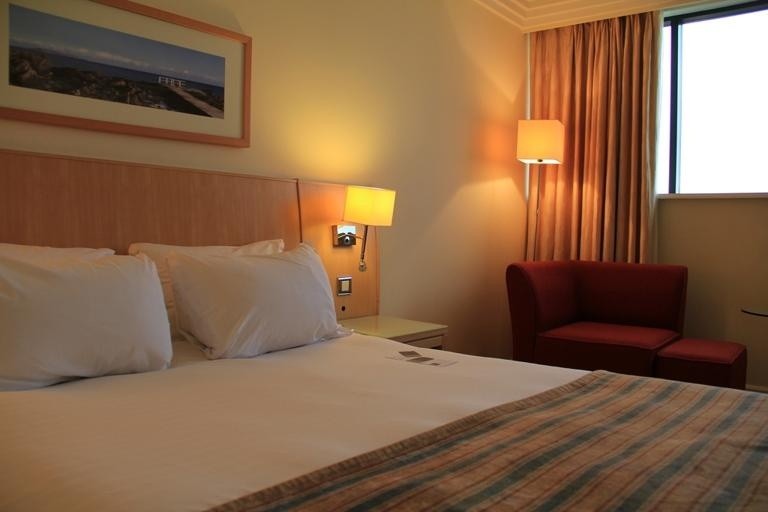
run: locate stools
[657,336,748,389]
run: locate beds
[2,149,766,510]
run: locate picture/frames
[0,0,257,149]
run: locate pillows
[1,248,175,391]
[0,241,116,255]
[125,233,287,344]
[165,240,353,362]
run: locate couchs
[504,259,691,379]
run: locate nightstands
[342,311,452,354]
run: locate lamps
[341,181,398,272]
[515,117,567,263]
